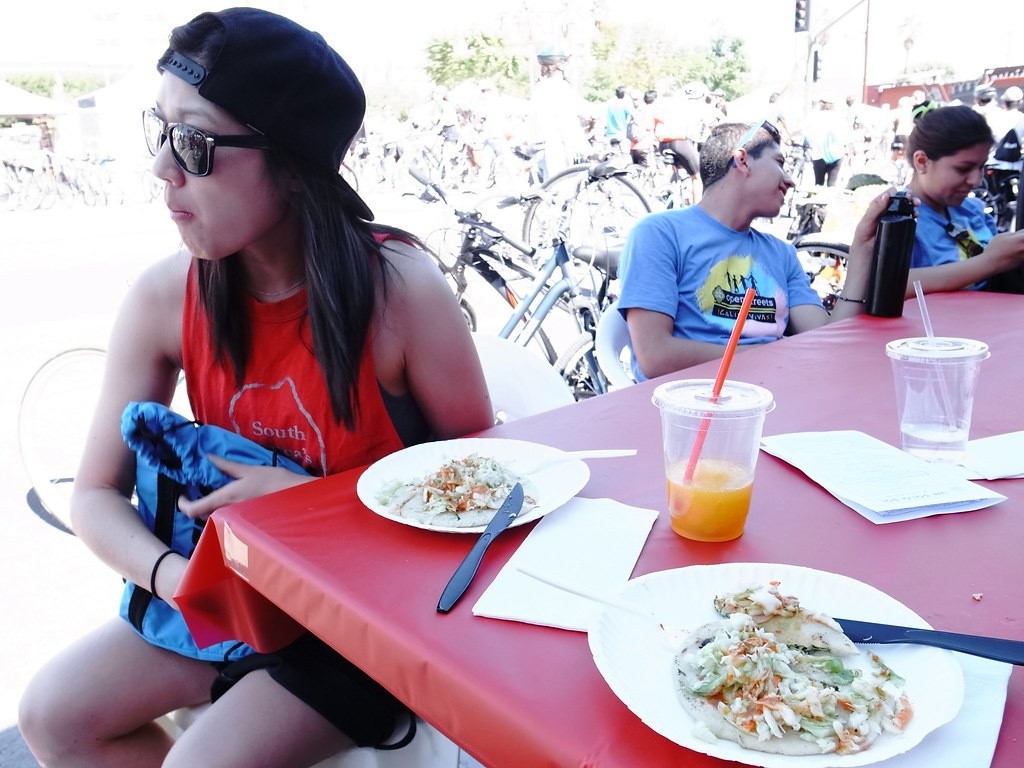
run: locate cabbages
[682,581,902,752]
[415,456,511,519]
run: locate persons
[12,6,493,768]
[613,123,922,381]
[609,87,927,185]
[892,102,1024,299]
[966,84,1024,226]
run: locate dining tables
[177,289,1024,768]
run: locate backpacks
[994,115,1021,162]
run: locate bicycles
[2,106,1024,404]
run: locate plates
[356,436,591,534]
[587,562,964,768]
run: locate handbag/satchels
[120,399,314,663]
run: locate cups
[651,377,776,544]
[884,335,991,468]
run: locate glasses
[142,109,279,177]
[732,121,782,166]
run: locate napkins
[476,497,660,633]
[966,430,1024,482]
[861,650,1013,768]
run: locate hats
[1000,86,1023,102]
[159,6,374,219]
[975,85,996,100]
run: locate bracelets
[839,295,866,305]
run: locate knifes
[832,617,1024,667]
[436,481,525,614]
